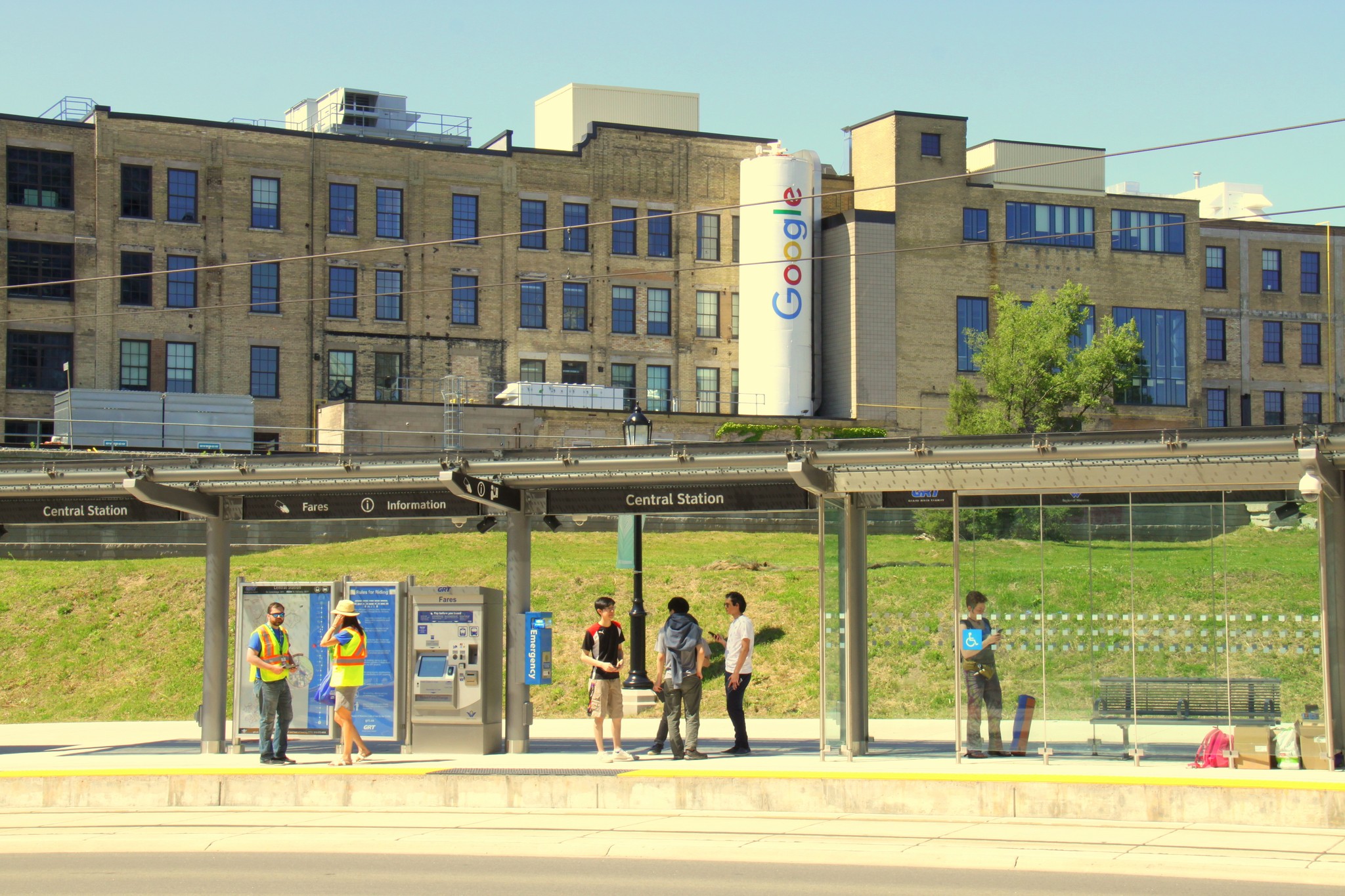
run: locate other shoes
[673,751,685,759]
[720,746,738,754]
[648,749,662,755]
[735,747,751,756]
[275,756,296,764]
[260,758,284,765]
[684,749,708,760]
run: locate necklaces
[968,616,981,625]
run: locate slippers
[327,758,353,766]
[353,752,372,763]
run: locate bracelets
[332,626,336,629]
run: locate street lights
[621,399,653,690]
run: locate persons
[714,591,755,756]
[959,591,1004,759]
[653,597,708,760]
[647,613,712,755]
[581,597,639,762]
[246,603,297,765]
[320,600,372,767]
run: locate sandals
[967,749,988,758]
[988,748,1012,756]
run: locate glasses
[270,612,285,617]
[602,606,616,611]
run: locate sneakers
[611,750,639,761]
[596,751,613,763]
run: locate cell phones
[708,632,719,639]
[997,629,1003,634]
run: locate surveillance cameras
[451,517,466,527]
[572,514,587,525]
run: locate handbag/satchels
[1272,728,1302,769]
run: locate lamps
[477,517,496,535]
[543,514,563,534]
[452,517,467,527]
[572,515,587,526]
[712,347,717,355]
[1298,466,1323,502]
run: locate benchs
[1090,676,1281,760]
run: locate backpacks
[1197,728,1235,767]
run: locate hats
[330,600,360,617]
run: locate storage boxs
[1230,724,1272,770]
[1294,719,1329,770]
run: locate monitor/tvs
[417,656,447,678]
